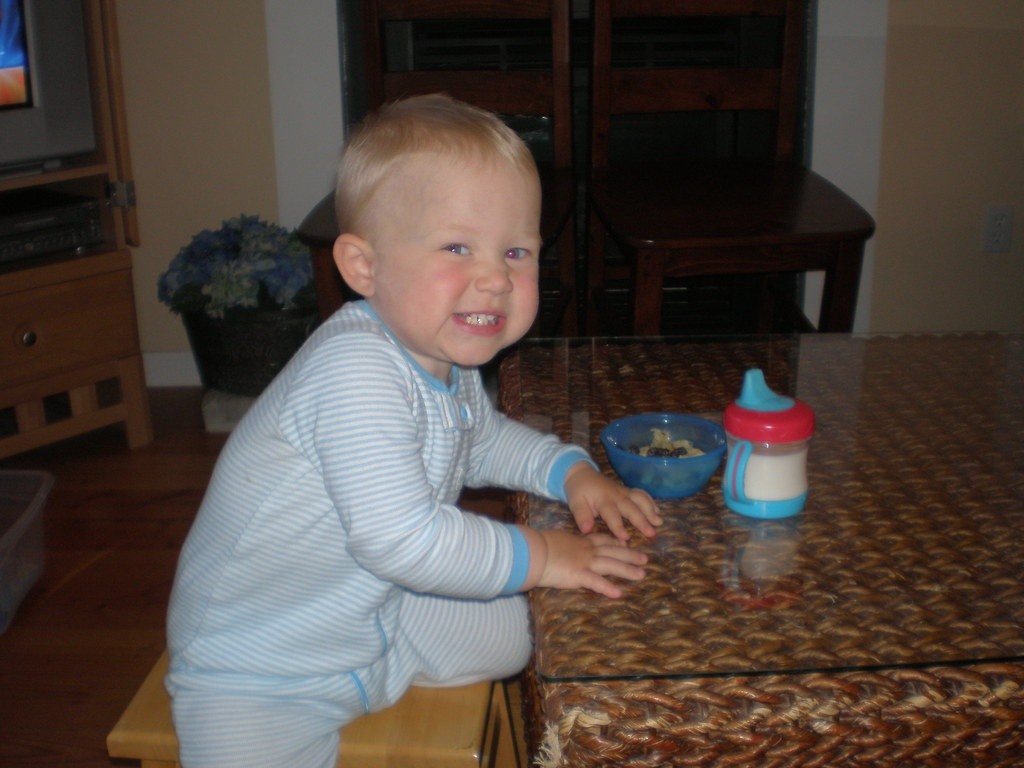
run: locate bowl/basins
[599,413,728,499]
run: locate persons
[165,93,663,768]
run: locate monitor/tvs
[0,0,97,181]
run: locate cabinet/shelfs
[0,0,154,464]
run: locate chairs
[288,0,575,337]
[585,0,878,339]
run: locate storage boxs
[0,463,59,636]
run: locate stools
[107,641,522,768]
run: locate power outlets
[981,205,1016,256]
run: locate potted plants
[156,210,310,399]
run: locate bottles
[722,369,814,518]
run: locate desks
[496,322,1024,768]
